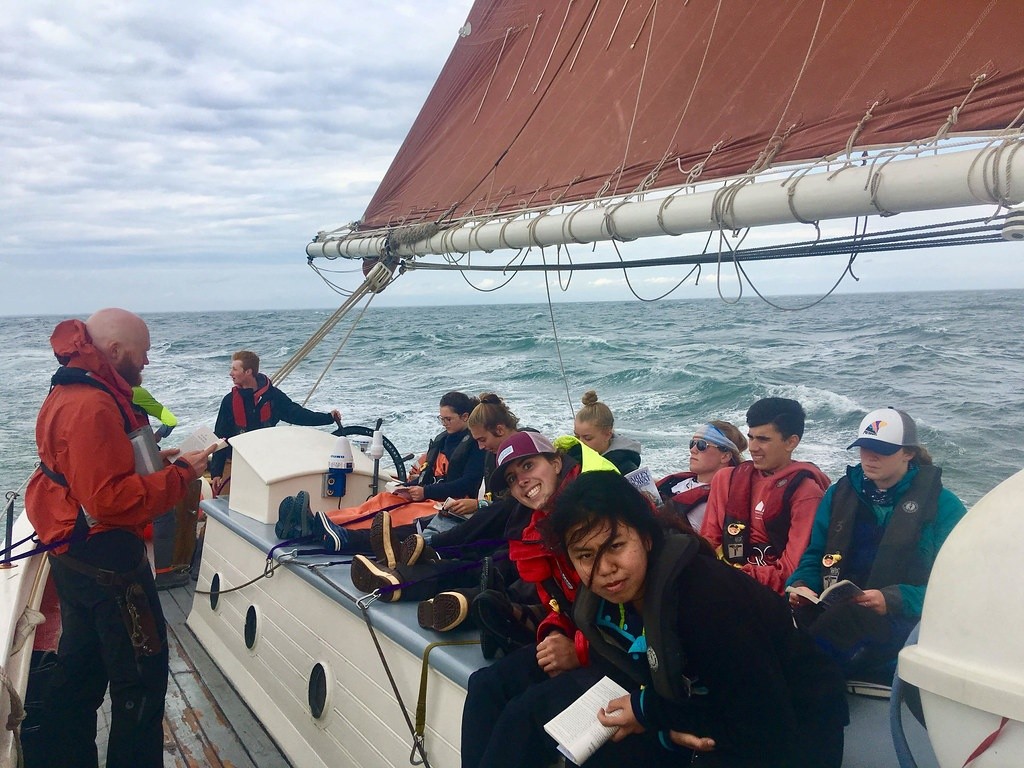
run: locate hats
[490,430,558,492]
[846,406,919,456]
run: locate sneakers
[369,511,402,571]
[418,591,468,632]
[401,534,440,565]
[351,555,406,603]
[154,571,189,591]
[316,511,350,553]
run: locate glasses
[689,439,726,452]
[437,414,462,425]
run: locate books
[167,426,227,466]
[433,497,472,520]
[623,465,664,508]
[386,482,411,501]
[784,579,865,611]
[543,676,632,766]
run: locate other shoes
[293,490,315,540]
[477,590,539,647]
[476,557,507,659]
[275,495,297,539]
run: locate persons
[210,350,341,500]
[23,308,216,768]
[131,386,177,443]
[278,391,967,768]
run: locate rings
[865,602,867,607]
